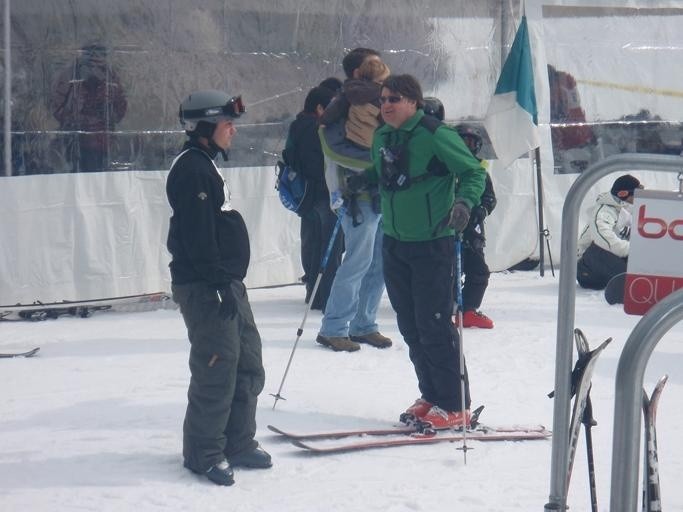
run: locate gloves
[447,202,470,234]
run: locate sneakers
[185,452,234,483]
[349,333,393,348]
[456,309,493,329]
[229,445,272,468]
[406,398,471,430]
[316,334,360,352]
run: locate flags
[484,12,543,168]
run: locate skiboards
[642,375,668,512]
[562,328,612,512]
[267,425,554,452]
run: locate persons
[346,73,488,433]
[167,88,274,485]
[281,48,395,350]
[548,64,591,165]
[417,95,493,330]
[53,33,128,172]
[577,174,644,290]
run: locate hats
[611,175,643,199]
[358,56,392,84]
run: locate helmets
[422,97,443,120]
[179,89,235,133]
[454,124,482,156]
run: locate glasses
[179,94,247,118]
[378,94,405,105]
